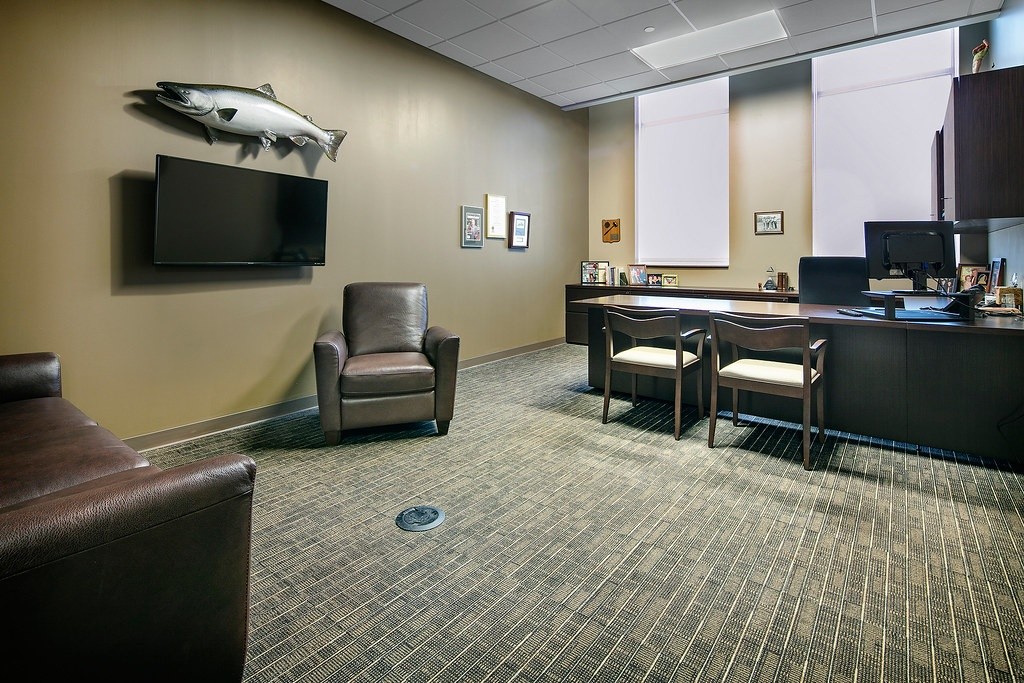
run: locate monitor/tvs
[864,221,956,294]
[153,154,328,267]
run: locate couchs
[0,350,256,683]
[313,281,460,445]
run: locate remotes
[837,308,863,317]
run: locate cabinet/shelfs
[564,283,798,346]
[931,64,1024,235]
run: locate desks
[571,295,1024,477]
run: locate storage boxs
[995,287,1022,305]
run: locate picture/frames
[647,273,662,286]
[461,204,485,248]
[581,261,610,284]
[986,257,1005,293]
[956,263,990,294]
[937,273,958,293]
[753,210,784,235]
[662,274,679,287]
[508,211,531,249]
[627,263,648,285]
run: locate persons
[466,218,479,241]
[648,275,662,284]
[962,267,987,290]
[761,216,778,230]
[633,268,643,284]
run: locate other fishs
[156,81,348,163]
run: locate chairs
[601,305,708,441]
[797,256,905,308]
[706,311,828,471]
